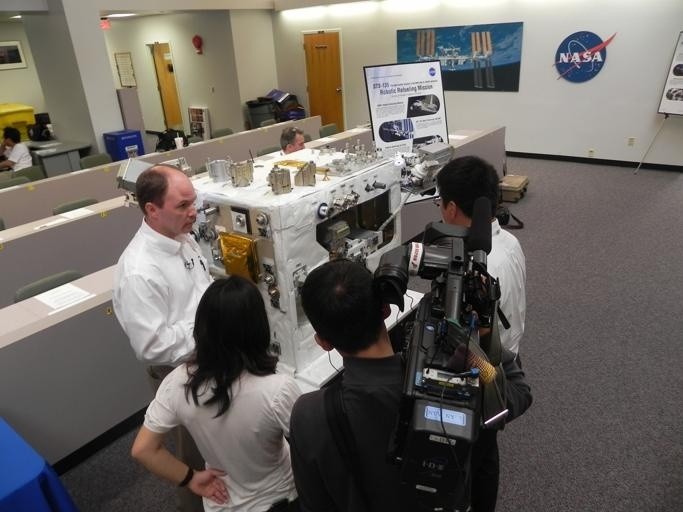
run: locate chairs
[0,118,342,303]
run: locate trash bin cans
[246,100,280,129]
[103,130,144,162]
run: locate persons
[431,156,526,511]
[280,127,305,156]
[131,275,305,511]
[112,164,216,512]
[0,128,33,172]
[288,258,532,512]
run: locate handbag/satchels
[27,123,50,141]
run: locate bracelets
[179,467,194,488]
